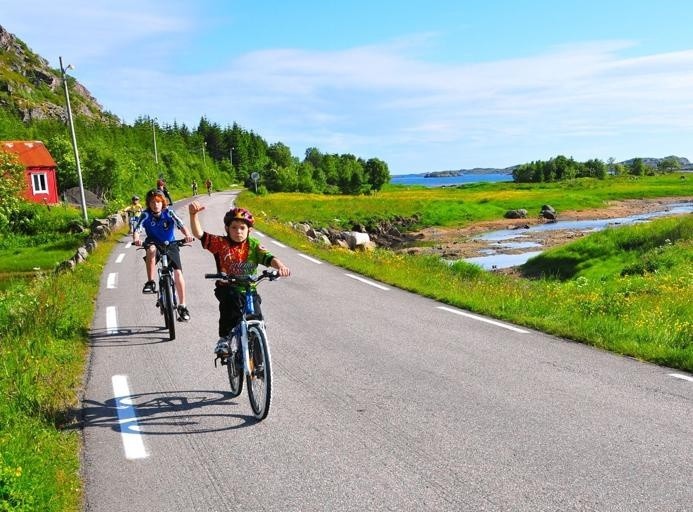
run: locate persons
[155,175,169,196]
[132,188,194,322]
[191,179,197,196]
[187,200,291,381]
[204,178,212,192]
[122,194,142,235]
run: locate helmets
[224,207,255,227]
[146,188,166,205]
[132,195,139,201]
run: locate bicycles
[131,238,195,340]
[193,189,198,196]
[207,185,212,196]
[124,210,140,233]
[204,270,291,421]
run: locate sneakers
[214,339,230,354]
[176,306,190,320]
[143,281,156,294]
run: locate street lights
[202,142,208,165]
[59,56,89,223]
[229,147,235,165]
[152,116,158,163]
